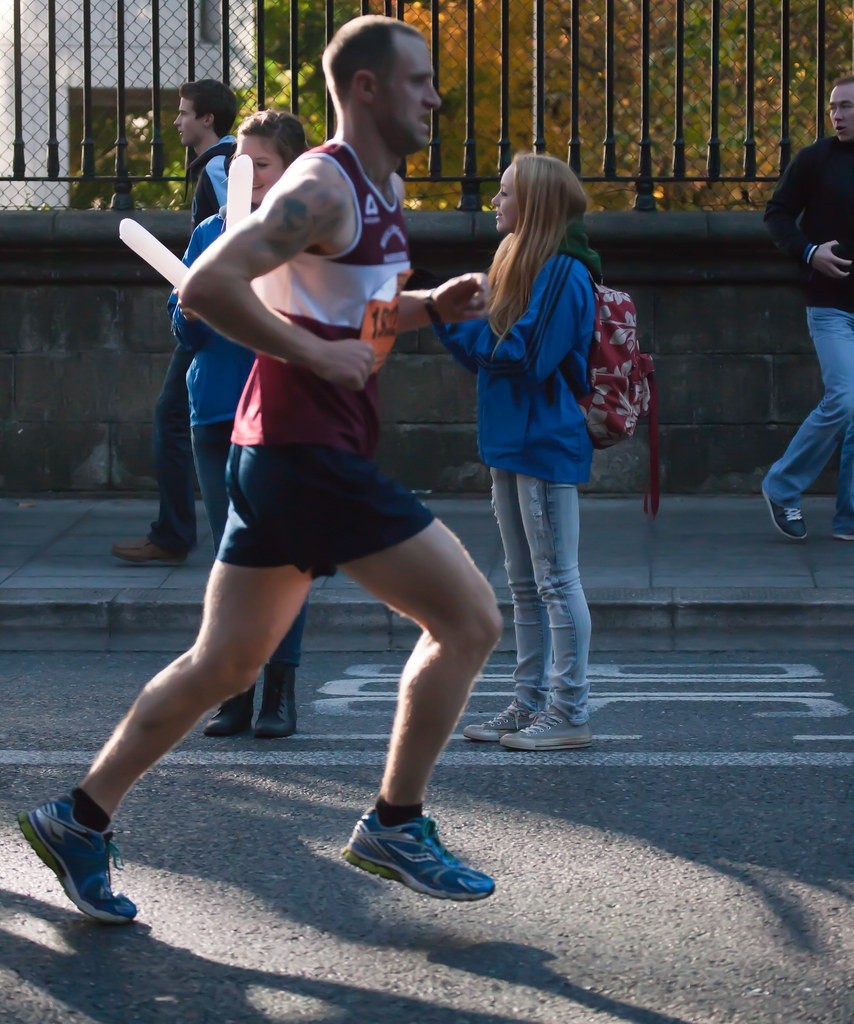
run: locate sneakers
[340,806,495,901]
[762,482,807,539]
[18,794,137,923]
[500,704,592,750]
[833,534,854,540]
[463,699,540,741]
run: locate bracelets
[423,288,444,329]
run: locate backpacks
[562,268,655,450]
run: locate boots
[203,683,255,736]
[255,661,297,736]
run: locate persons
[761,76,854,539]
[402,152,603,749]
[14,14,504,924]
[166,111,314,739]
[110,81,237,565]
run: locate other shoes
[112,537,188,565]
